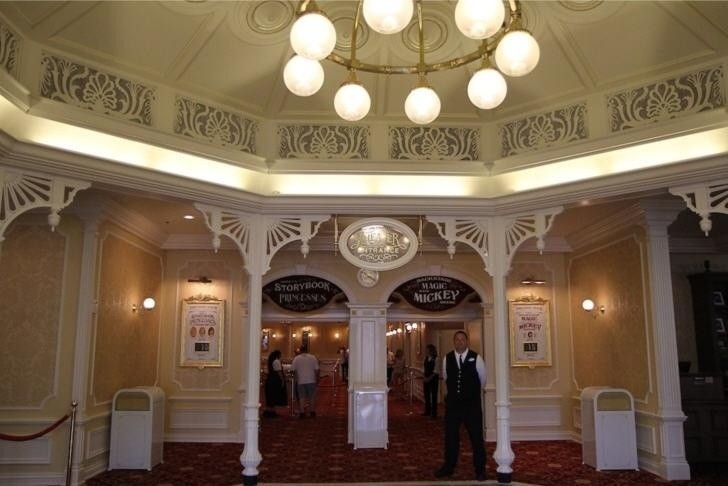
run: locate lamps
[282,0,539,125]
[188,277,212,283]
[522,279,547,285]
[132,298,155,312]
[582,300,605,314]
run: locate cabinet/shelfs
[686,260,728,375]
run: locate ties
[458,354,462,370]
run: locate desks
[681,374,728,470]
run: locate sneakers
[475,470,487,481]
[433,465,454,478]
[299,410,315,418]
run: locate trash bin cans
[581,386,640,472]
[107,386,162,471]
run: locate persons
[420,344,440,418]
[433,330,489,482]
[262,350,288,418]
[333,344,348,384]
[386,346,396,385]
[391,349,405,385]
[290,346,320,419]
